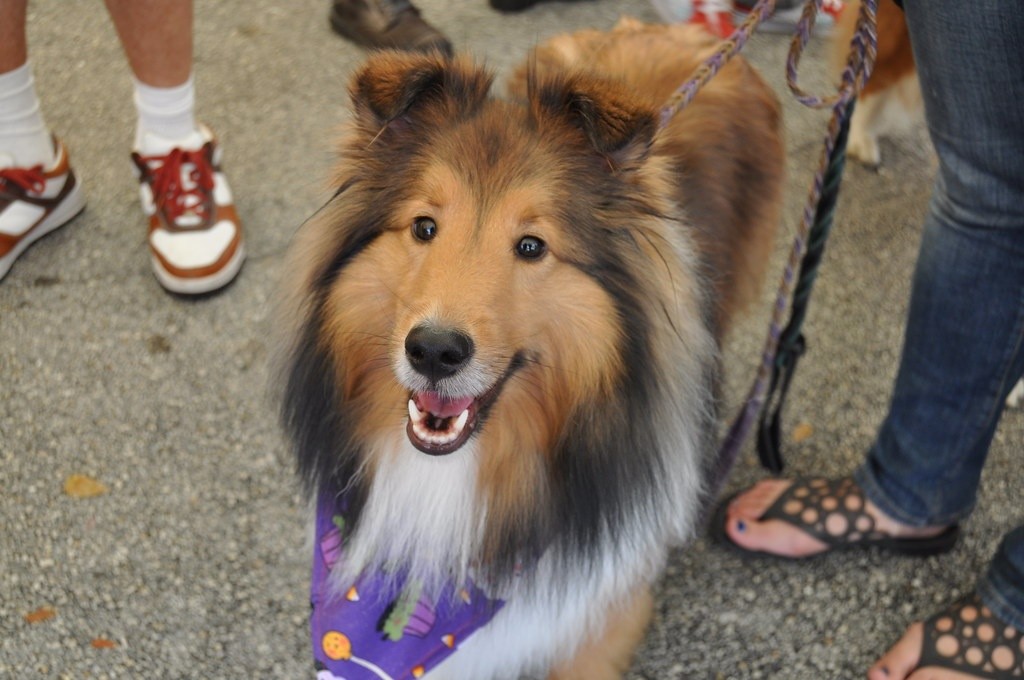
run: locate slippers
[709,474,961,559]
[864,589,1023,680]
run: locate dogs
[827,1,1024,411]
[258,11,784,680]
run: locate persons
[1,0,248,295]
[704,0,1024,680]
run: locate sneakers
[1,132,84,285]
[731,5,836,35]
[130,115,248,293]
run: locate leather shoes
[331,0,453,60]
